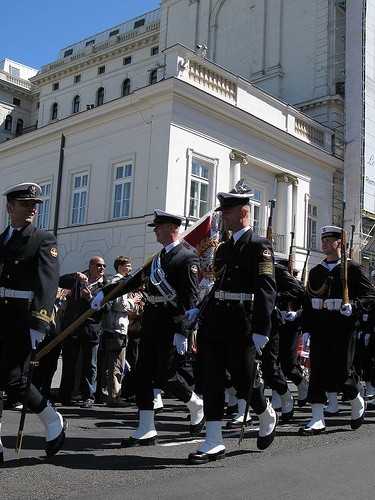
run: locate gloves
[284,311,297,322]
[365,334,370,346]
[253,333,269,355]
[173,333,188,355]
[340,303,352,316]
[185,308,199,320]
[30,329,46,349]
[91,290,104,311]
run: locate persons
[3,192,375,462]
[0,183,68,463]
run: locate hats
[319,226,347,239]
[147,209,184,227]
[1,182,44,204]
[214,192,254,212]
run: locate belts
[0,287,35,299]
[215,290,254,303]
[147,295,169,302]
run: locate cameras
[80,278,88,282]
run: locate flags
[183,208,225,306]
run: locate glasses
[94,263,107,268]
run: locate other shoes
[121,435,159,448]
[257,410,279,451]
[45,412,68,457]
[190,416,205,438]
[188,448,226,465]
[224,386,375,436]
[14,396,137,413]
[137,405,164,416]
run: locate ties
[160,248,166,258]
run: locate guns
[15,286,71,453]
[349,223,356,261]
[288,213,296,277]
[341,200,350,312]
[264,199,275,246]
[301,249,312,288]
